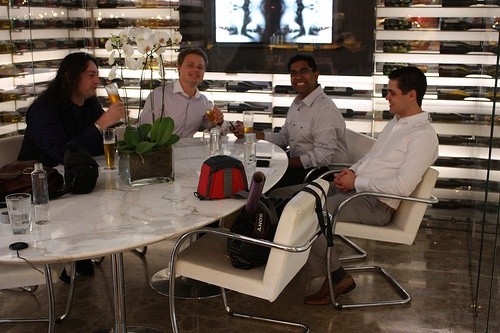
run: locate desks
[0,131,289,333]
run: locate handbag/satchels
[226,196,291,270]
[64,139,99,194]
[193,154,249,200]
[0,159,65,210]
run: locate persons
[140,47,228,138]
[17,52,125,278]
[266,66,438,304]
[231,56,349,197]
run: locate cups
[243,142,257,184]
[103,128,117,170]
[243,110,254,143]
[210,128,222,156]
[5,193,33,235]
[222,128,229,156]
[105,82,127,119]
[203,91,217,126]
[203,133,214,161]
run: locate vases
[114,142,176,187]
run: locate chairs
[0,135,75,323]
[168,178,332,333]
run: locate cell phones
[256,160,270,168]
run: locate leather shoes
[304,272,357,305]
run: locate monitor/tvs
[211,0,338,49]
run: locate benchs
[322,128,439,309]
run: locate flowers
[105,25,182,153]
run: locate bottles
[273,106,290,115]
[31,163,51,224]
[0,0,179,141]
[336,109,366,118]
[274,84,298,94]
[374,0,500,194]
[227,102,268,112]
[324,85,367,96]
[226,79,267,92]
[196,80,223,90]
